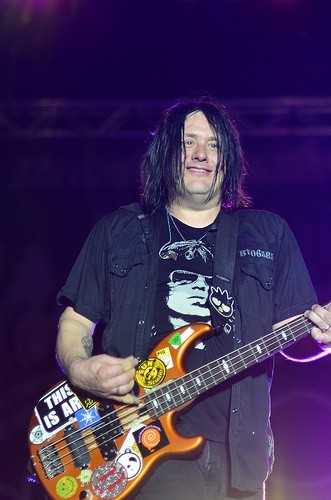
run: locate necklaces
[164,204,210,260]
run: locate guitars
[27,302,331,500]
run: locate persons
[53,97,331,500]
